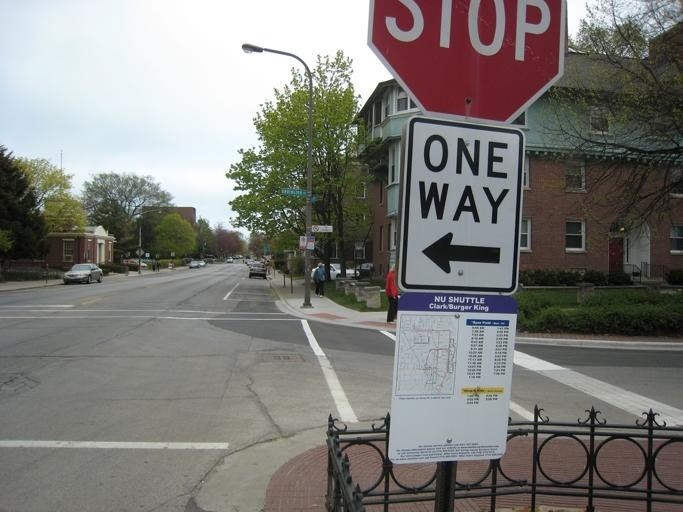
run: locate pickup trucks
[204,255,216,264]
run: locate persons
[312,263,327,298]
[155,262,161,272]
[384,263,399,324]
[152,258,156,272]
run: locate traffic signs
[395,116,525,295]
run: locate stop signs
[367,0,568,126]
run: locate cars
[121,259,146,271]
[64,263,103,284]
[189,261,205,269]
[227,255,243,263]
[245,259,266,279]
[311,262,373,279]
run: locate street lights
[171,251,176,269]
[138,209,162,274]
[242,44,313,308]
